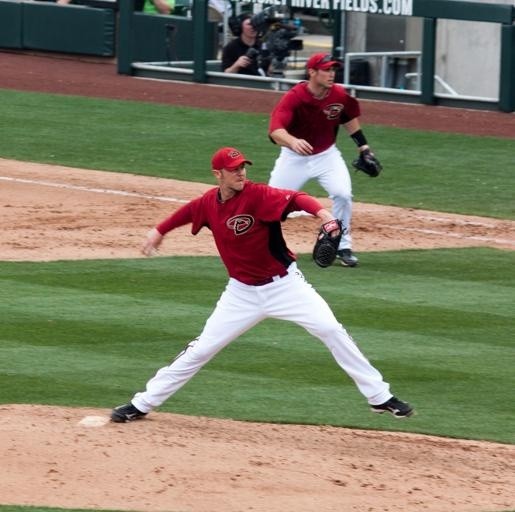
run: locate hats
[305,52,345,72]
[211,146,253,173]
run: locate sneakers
[109,399,148,423]
[369,393,414,420]
[335,248,360,267]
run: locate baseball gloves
[312,219,342,267]
[352,148,382,176]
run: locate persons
[111,147,414,423]
[221,13,273,76]
[266,54,369,267]
[144,0,176,16]
